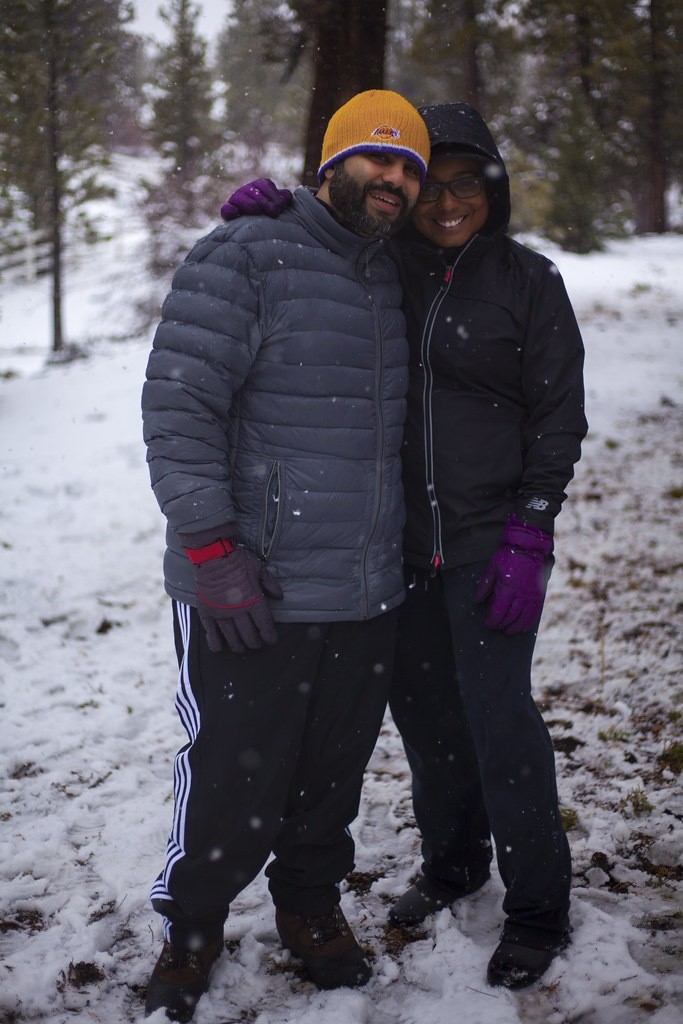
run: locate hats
[316,89,430,191]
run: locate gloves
[220,179,291,221]
[178,521,282,654]
[474,515,553,635]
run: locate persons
[140,89,588,1022]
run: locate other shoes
[486,919,570,994]
[145,928,225,1022]
[276,902,372,992]
[388,876,488,926]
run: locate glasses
[417,176,484,203]
